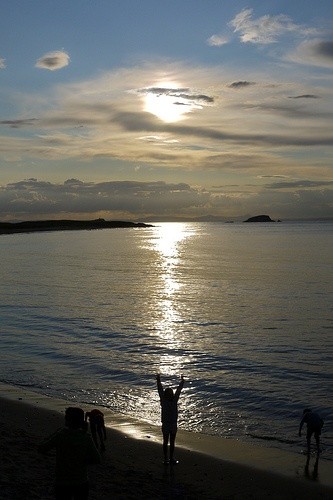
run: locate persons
[45,407,103,500]
[156,372,184,465]
[299,408,323,449]
